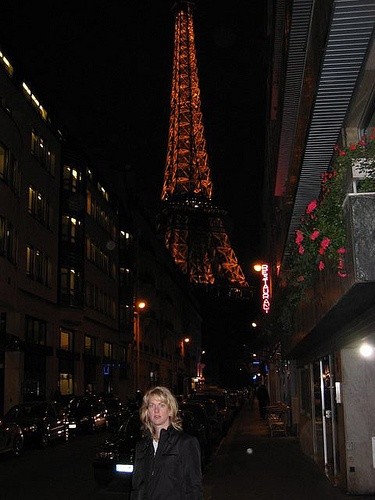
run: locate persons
[128,385,206,500]
[247,383,270,419]
[53,386,62,403]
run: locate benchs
[263,401,290,438]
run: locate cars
[0,417,25,457]
[4,401,73,450]
[96,388,249,487]
[59,394,109,434]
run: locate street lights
[135,299,146,391]
[181,336,190,395]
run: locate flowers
[267,128,374,293]
